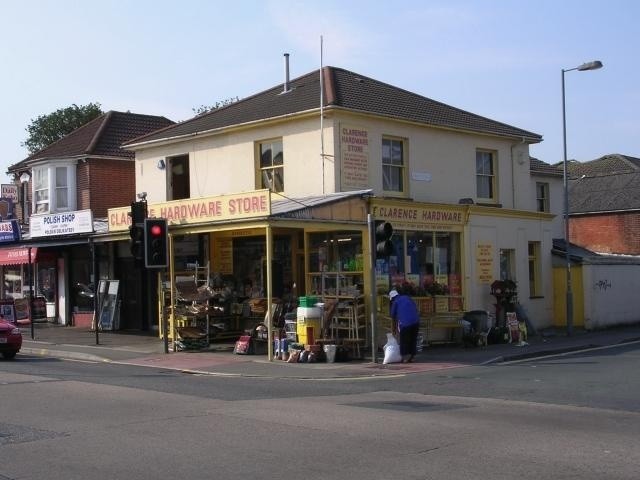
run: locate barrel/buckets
[323,344,336,362]
[464,310,488,348]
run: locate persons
[388,289,421,364]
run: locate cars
[0,315,23,361]
[75,282,96,310]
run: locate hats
[388,290,401,301]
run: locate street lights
[560,59,604,336]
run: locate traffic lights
[374,219,394,259]
[144,218,170,268]
[128,202,147,260]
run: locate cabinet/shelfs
[156,267,367,359]
